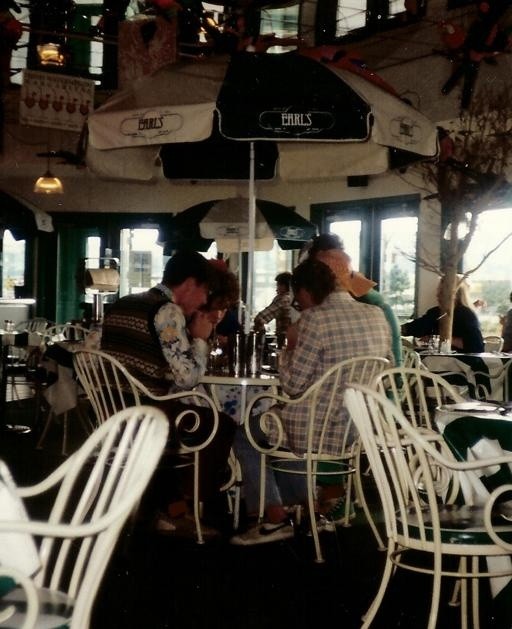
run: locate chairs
[482,335,505,353]
[371,366,467,449]
[3,315,105,460]
[242,357,395,565]
[469,358,512,403]
[0,403,168,629]
[402,345,471,410]
[363,348,422,476]
[70,345,243,545]
[342,377,511,629]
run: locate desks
[432,400,511,607]
[0,458,42,606]
[191,373,282,531]
[414,351,512,406]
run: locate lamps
[32,127,66,196]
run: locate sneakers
[229,519,295,546]
[157,510,218,536]
[302,492,355,538]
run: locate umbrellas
[153,198,316,327]
[1,189,57,242]
[80,51,444,345]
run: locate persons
[498,291,512,403]
[400,273,492,400]
[98,233,405,545]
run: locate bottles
[3,319,16,334]
[428,334,451,355]
[229,330,263,376]
